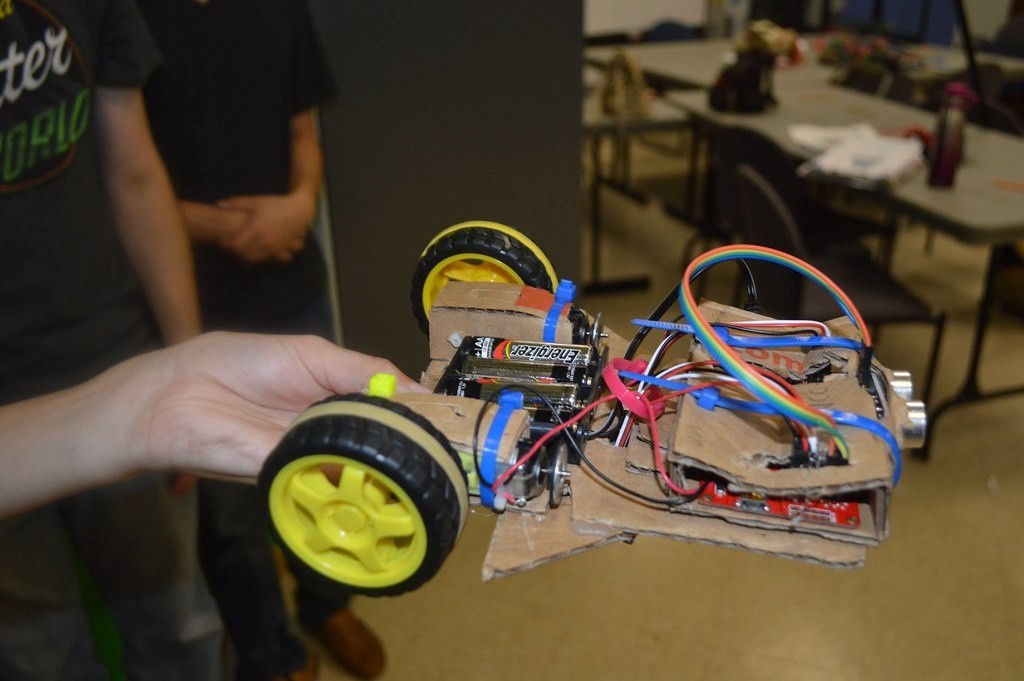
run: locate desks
[575,33,1024,468]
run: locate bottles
[930,81,966,189]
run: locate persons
[0,330,432,521]
[87,1,386,681]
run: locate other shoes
[290,647,318,681]
[296,609,386,681]
